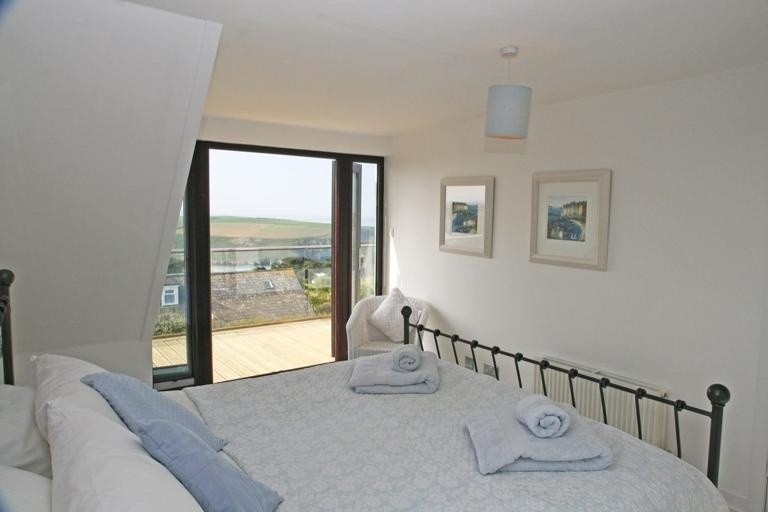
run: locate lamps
[485,47,532,140]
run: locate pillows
[139,419,284,511]
[368,288,421,343]
[27,353,131,445]
[81,372,227,454]
[2,383,52,477]
[0,465,51,512]
[42,401,203,512]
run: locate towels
[515,394,570,438]
[465,402,613,476]
[390,344,421,373]
[347,351,439,394]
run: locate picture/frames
[439,176,494,259]
[529,170,612,272]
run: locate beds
[0,269,733,512]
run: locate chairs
[345,295,430,360]
